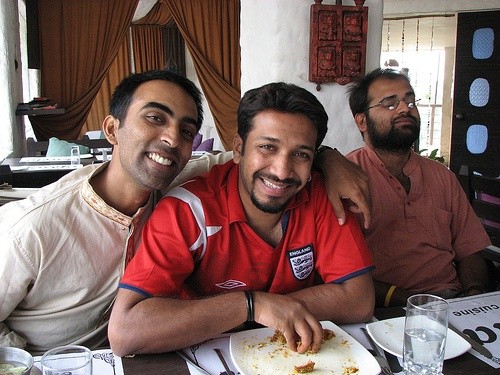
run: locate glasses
[362,95,420,110]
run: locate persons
[104,82,375,358]
[0,70,371,358]
[344,67,491,308]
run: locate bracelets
[315,146,338,164]
[244,290,254,321]
[384,285,396,307]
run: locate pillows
[46,136,89,158]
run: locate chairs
[25,135,89,156]
[87,136,113,154]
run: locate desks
[1,156,102,184]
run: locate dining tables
[33,282,500,375]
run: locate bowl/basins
[0,346,34,375]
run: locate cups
[41,345,93,375]
[402,294,449,375]
[70,147,80,167]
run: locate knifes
[446,322,492,359]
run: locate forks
[359,327,394,375]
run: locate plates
[229,320,382,375]
[366,315,471,360]
